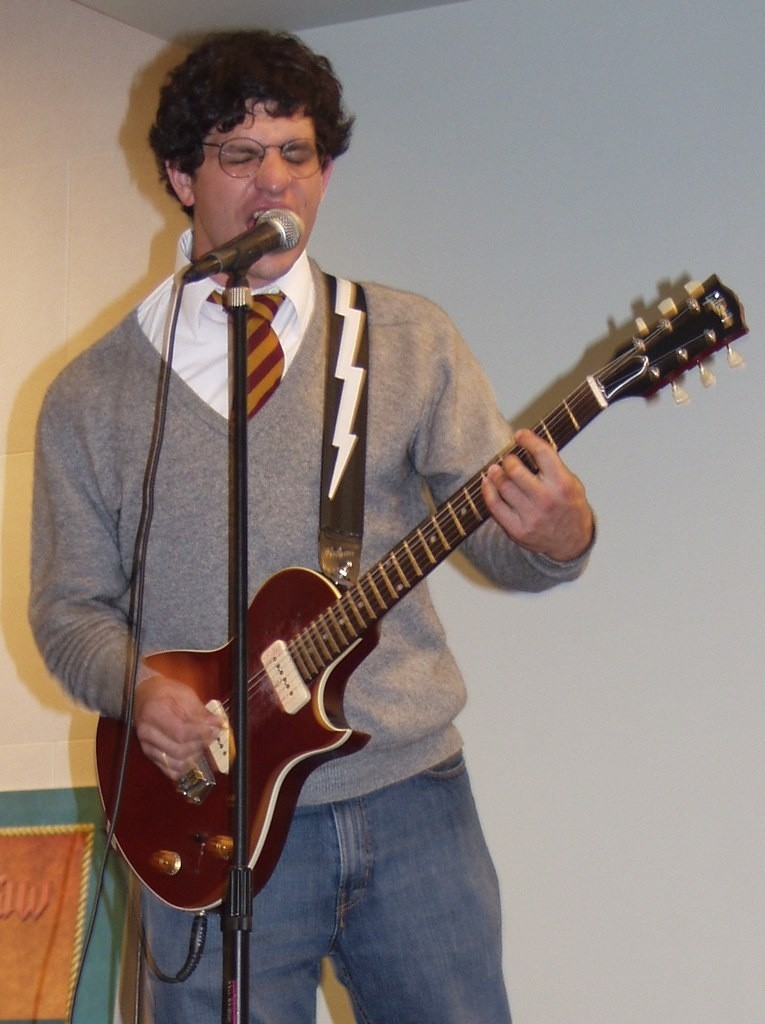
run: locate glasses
[199,137,329,179]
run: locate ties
[207,290,287,422]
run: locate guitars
[92,272,745,916]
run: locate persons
[30,32,597,1023]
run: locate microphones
[184,206,306,283]
